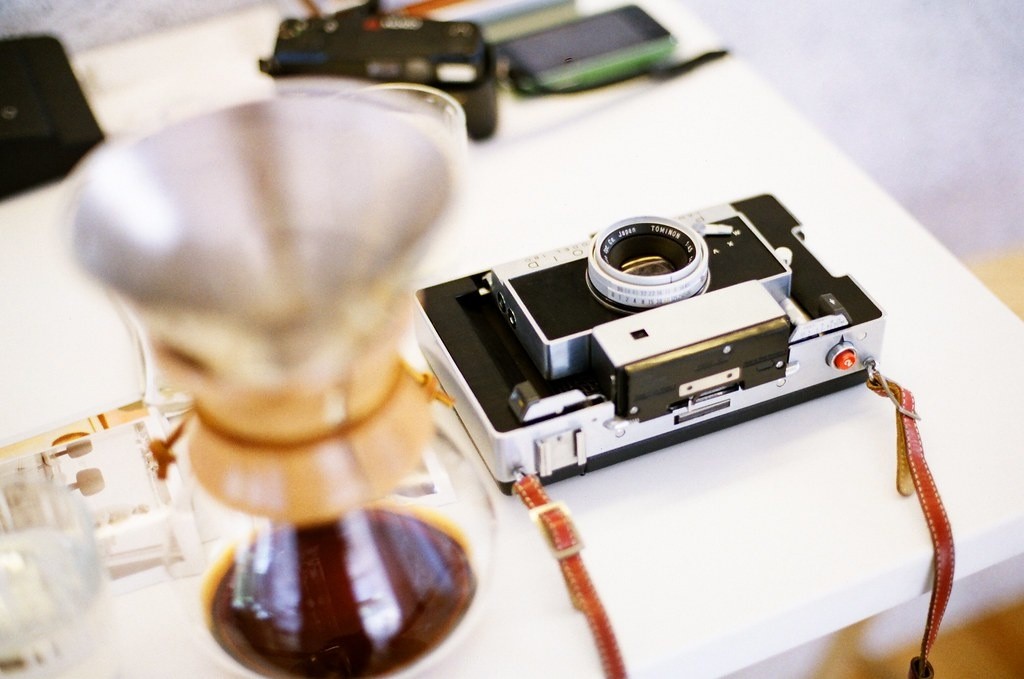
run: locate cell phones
[492,4,679,94]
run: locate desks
[0,0,1023,678]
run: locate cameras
[259,15,497,142]
[414,192,888,497]
